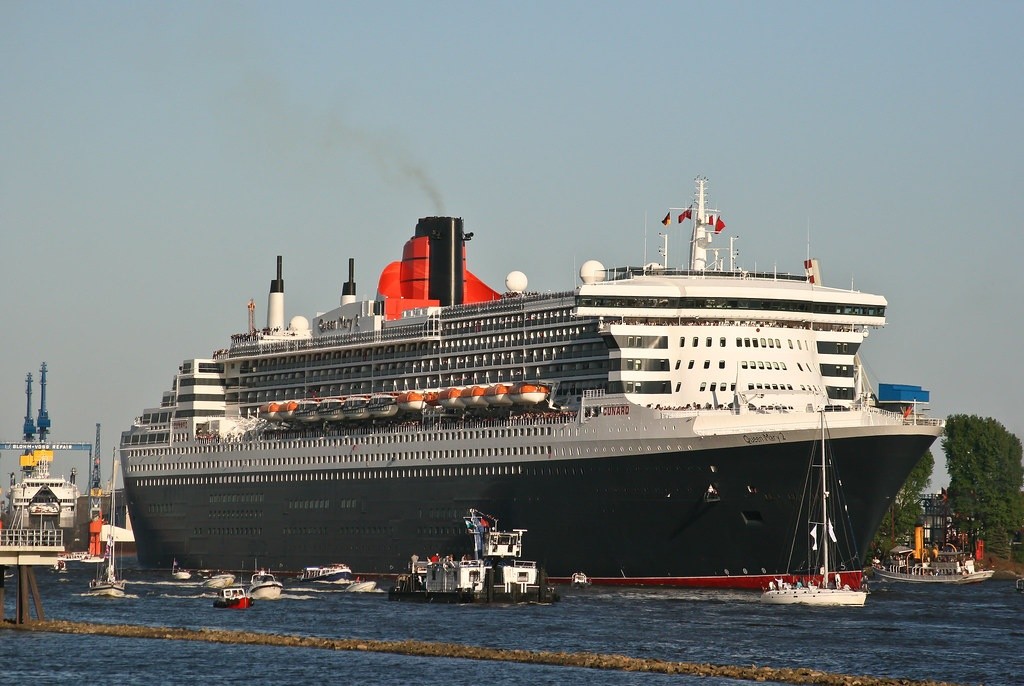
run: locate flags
[464,518,489,528]
[810,518,837,550]
[708,215,714,225]
[715,216,726,234]
[661,212,671,227]
[804,260,815,284]
[678,206,693,224]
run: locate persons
[500,291,539,299]
[262,410,578,439]
[581,298,861,333]
[212,326,280,359]
[646,404,724,410]
[872,530,976,576]
[774,572,869,591]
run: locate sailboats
[760,408,868,607]
[88,446,126,599]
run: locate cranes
[88,423,102,556]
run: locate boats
[212,576,254,610]
[300,563,353,583]
[53,551,105,571]
[571,572,592,590]
[248,558,283,600]
[871,521,996,585]
[5,361,81,545]
[388,509,562,607]
[344,580,377,592]
[196,560,236,589]
[171,558,192,580]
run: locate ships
[119,174,948,591]
